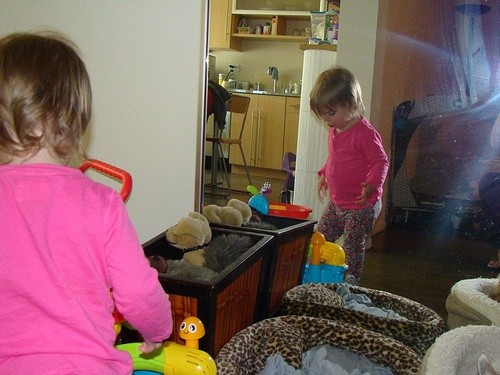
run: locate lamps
[454,3,492,97]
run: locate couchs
[386,93,500,231]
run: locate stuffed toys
[147,212,253,281]
[200,199,276,230]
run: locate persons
[0,33,171,375]
[310,68,390,286]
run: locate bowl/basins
[241,198,312,219]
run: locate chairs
[204,93,253,197]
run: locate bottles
[263,22,271,35]
[284,82,302,95]
[228,78,236,89]
[272,17,276,24]
[255,24,264,35]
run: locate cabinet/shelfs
[282,96,300,181]
[209,0,328,52]
[228,92,287,180]
